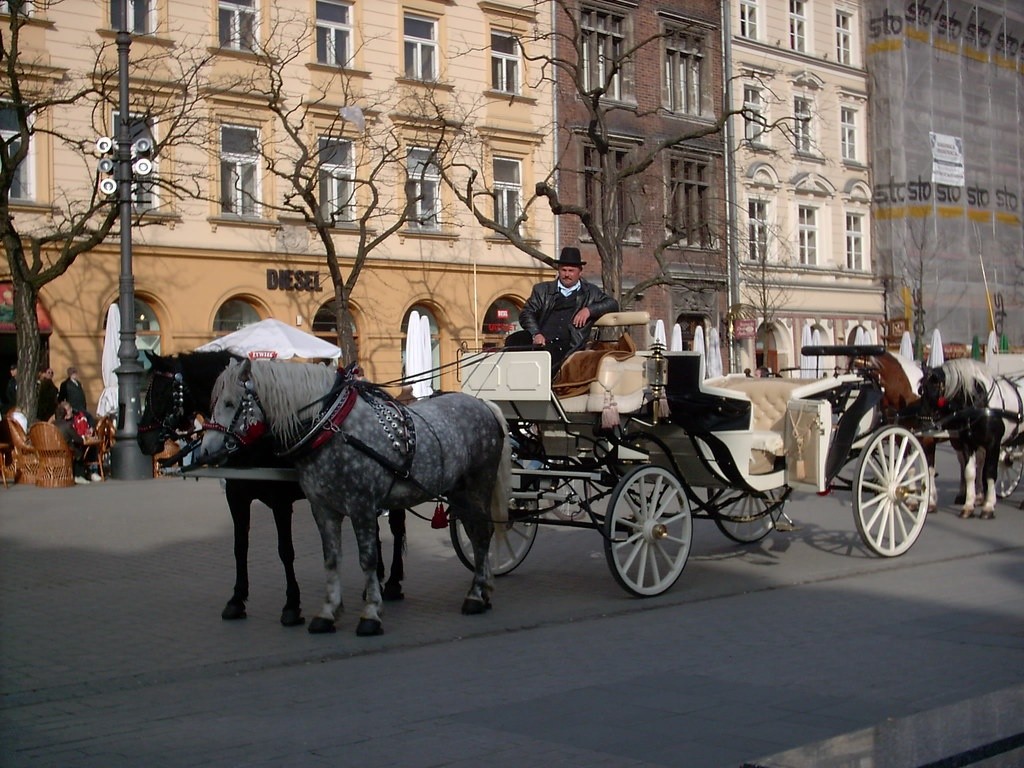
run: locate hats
[554,247,587,265]
[10,364,16,369]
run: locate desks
[0,443,11,489]
[84,440,102,458]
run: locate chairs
[7,411,39,486]
[29,421,76,490]
[152,438,179,480]
[83,414,117,481]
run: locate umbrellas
[801,324,824,379]
[196,316,342,363]
[707,327,723,378]
[988,331,999,358]
[899,331,913,360]
[926,329,944,367]
[854,327,871,345]
[98,303,122,428]
[693,325,705,379]
[671,323,682,351]
[654,319,666,351]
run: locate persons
[0,364,101,485]
[833,366,840,377]
[176,418,204,479]
[505,247,620,380]
[405,310,434,401]
[744,368,751,377]
[755,367,768,377]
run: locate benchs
[636,349,752,435]
[706,342,886,456]
[553,310,650,413]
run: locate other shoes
[74,476,90,485]
[86,473,101,481]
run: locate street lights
[97,1,157,482]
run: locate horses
[136,348,406,629]
[199,360,510,637]
[916,357,1024,520]
[846,352,984,512]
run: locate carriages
[135,310,932,637]
